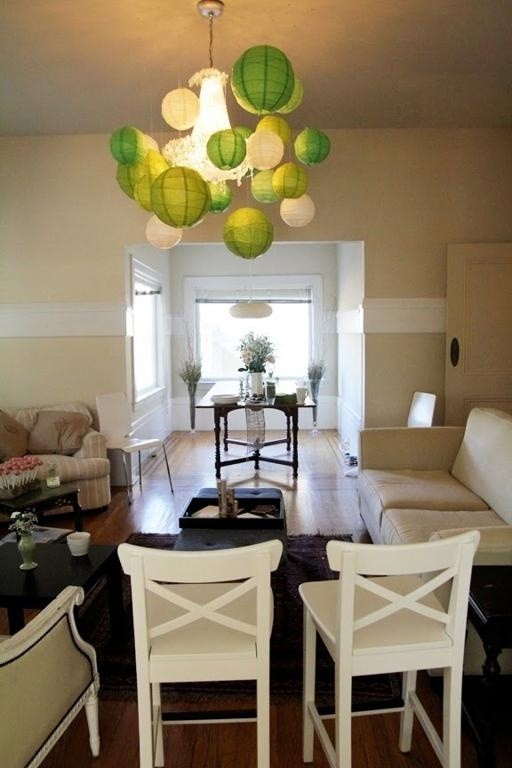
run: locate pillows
[0,408,90,462]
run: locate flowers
[236,329,276,372]
[12,505,37,536]
[0,456,44,475]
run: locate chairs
[114,539,283,768]
[296,529,481,768]
[406,391,437,427]
[0,584,105,768]
[95,390,176,504]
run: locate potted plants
[172,319,202,436]
[305,318,332,432]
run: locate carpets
[73,528,406,710]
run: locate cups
[67,531,91,557]
[296,388,309,403]
[350,456,358,466]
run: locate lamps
[229,304,272,319]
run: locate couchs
[0,399,113,514]
[355,405,512,566]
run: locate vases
[0,467,38,490]
[15,535,40,571]
[248,369,265,399]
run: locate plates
[211,394,240,404]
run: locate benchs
[171,485,286,554]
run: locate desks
[194,378,317,480]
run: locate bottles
[344,453,350,466]
